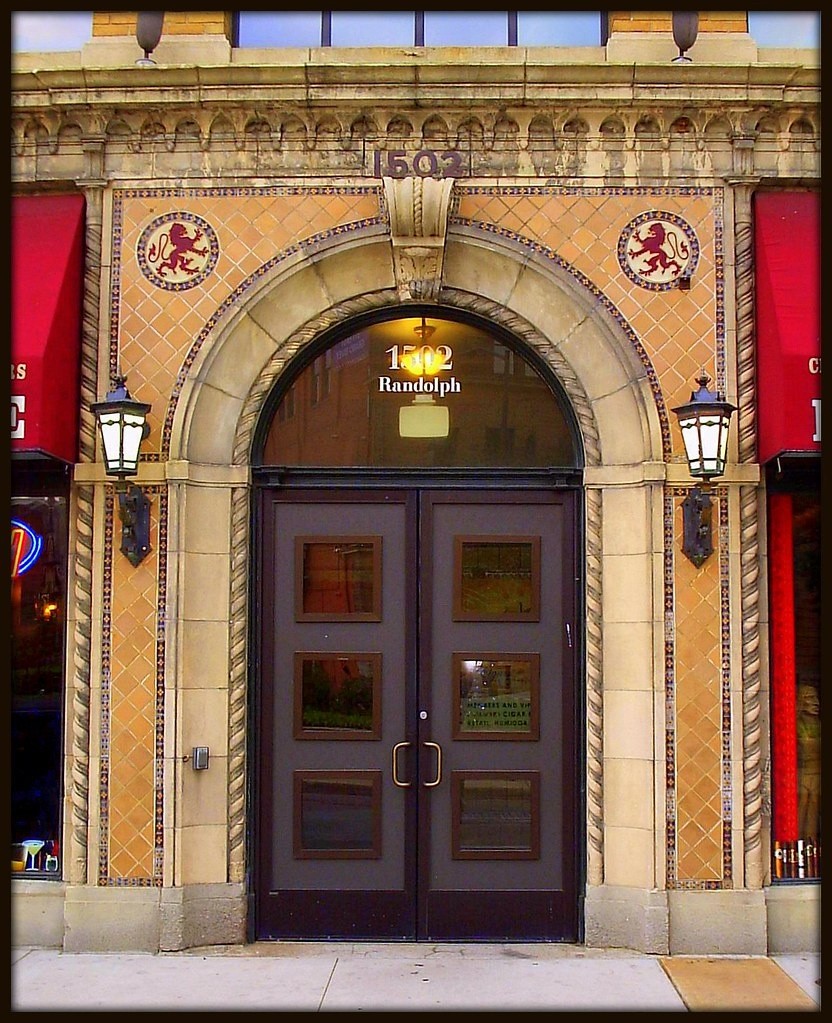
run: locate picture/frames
[294,535,384,622]
[293,767,383,861]
[450,650,542,742]
[452,533,543,623]
[292,649,384,742]
[449,768,541,861]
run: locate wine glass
[44,839,55,858]
[23,840,44,871]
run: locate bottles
[772,834,818,880]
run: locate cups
[11,842,28,872]
[45,855,59,872]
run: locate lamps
[397,314,450,438]
[669,366,740,568]
[402,325,449,377]
[90,366,152,569]
